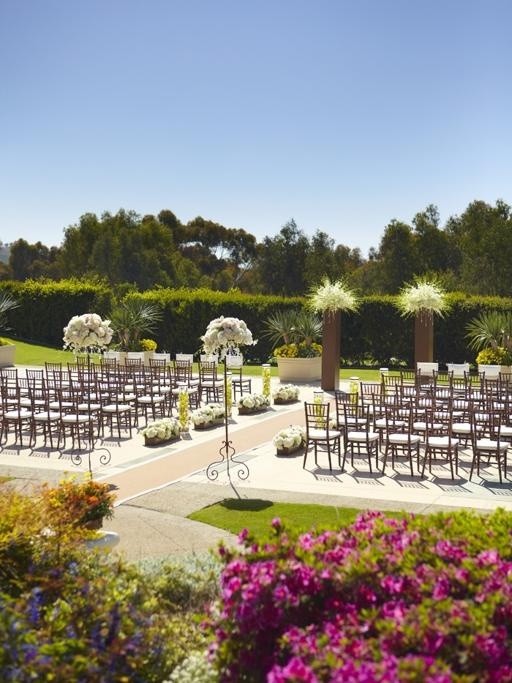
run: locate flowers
[304,271,363,320]
[200,315,259,355]
[0,472,512,682]
[273,385,299,400]
[102,294,164,350]
[259,310,322,357]
[237,393,271,411]
[392,273,451,323]
[62,312,115,355]
[464,308,512,365]
[272,425,302,454]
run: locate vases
[273,398,290,404]
[278,357,323,381]
[500,366,512,381]
[119,350,154,366]
[236,407,253,415]
[277,444,295,455]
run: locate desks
[417,362,502,378]
[103,352,243,369]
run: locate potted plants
[0,288,21,368]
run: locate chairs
[0,358,250,452]
[302,367,512,482]
